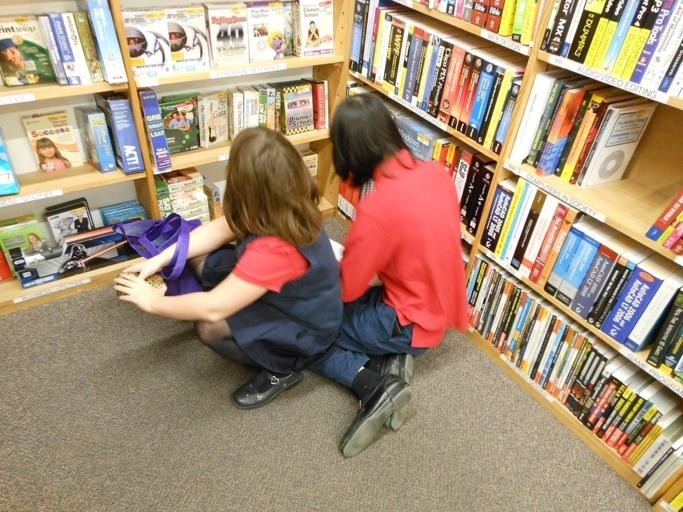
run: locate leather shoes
[228,368,304,409]
[338,353,412,457]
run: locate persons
[308,22,319,41]
[111,125,342,410]
[37,138,71,172]
[305,94,468,457]
[169,112,192,130]
[25,211,89,255]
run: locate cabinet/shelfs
[354,0,683,512]
[0,0,354,316]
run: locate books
[338,0,681,256]
[464,224,683,512]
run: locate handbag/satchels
[112,212,205,296]
